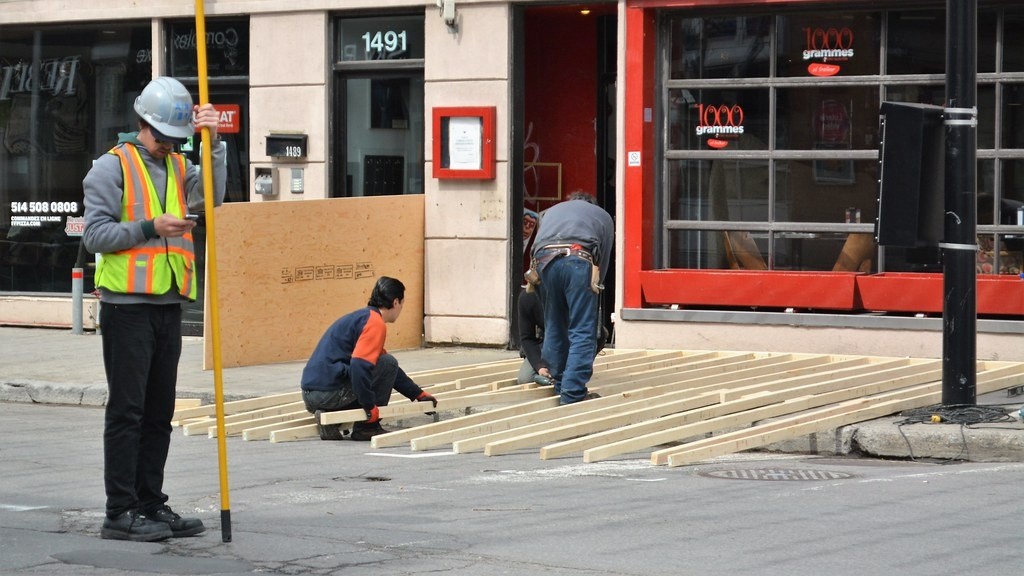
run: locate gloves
[365,405,380,423]
[417,390,437,408]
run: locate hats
[150,125,188,145]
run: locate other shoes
[583,393,602,402]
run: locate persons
[523,212,537,252]
[82,76,227,541]
[301,276,437,441]
[529,191,614,406]
[517,289,606,385]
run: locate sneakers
[315,409,343,440]
[350,417,391,441]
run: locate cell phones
[184,214,198,221]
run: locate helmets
[132,76,195,137]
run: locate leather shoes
[138,505,206,537]
[100,508,174,542]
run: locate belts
[541,245,593,272]
